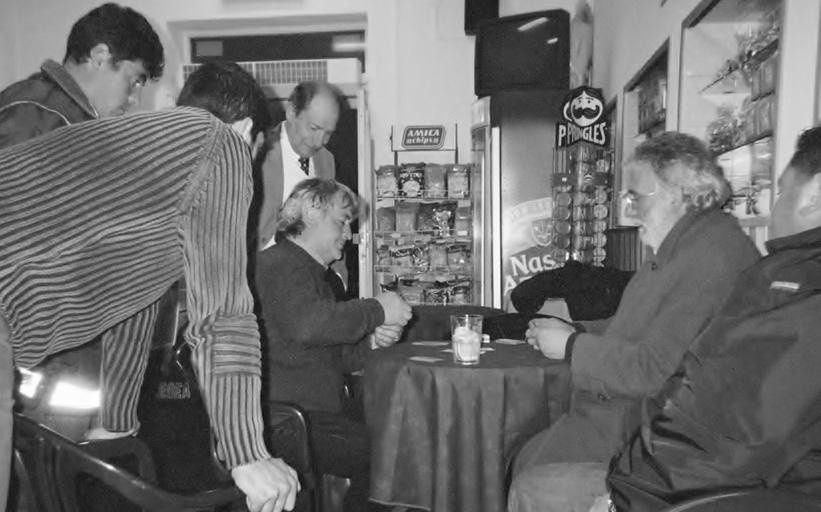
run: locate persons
[248,178,413,510]
[1,60,303,512]
[247,79,351,292]
[0,2,166,150]
[506,127,821,511]
[510,130,766,476]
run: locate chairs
[661,489,821,511]
[171,341,353,512]
[6,411,246,512]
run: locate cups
[450,314,484,366]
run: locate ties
[297,157,308,175]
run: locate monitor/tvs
[474,8,572,96]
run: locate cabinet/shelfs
[373,195,478,308]
[682,27,779,255]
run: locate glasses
[130,77,142,90]
[622,190,657,209]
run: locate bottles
[376,163,469,199]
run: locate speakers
[464,0,499,37]
